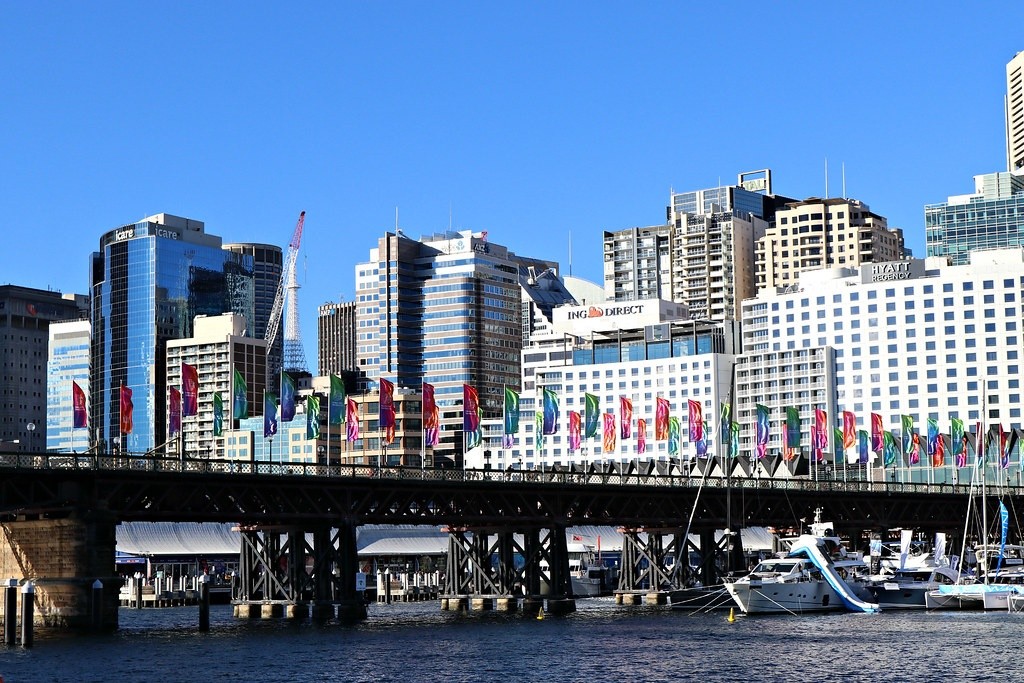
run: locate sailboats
[664,360,749,612]
[924,377,1024,611]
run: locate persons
[419,571,423,581]
[198,568,210,584]
[390,572,400,581]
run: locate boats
[864,529,959,611]
[520,543,613,599]
[726,508,875,615]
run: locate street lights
[517,454,523,479]
[749,456,761,489]
[317,446,325,463]
[27,423,35,469]
[822,458,899,482]
[382,440,389,465]
[267,434,274,474]
